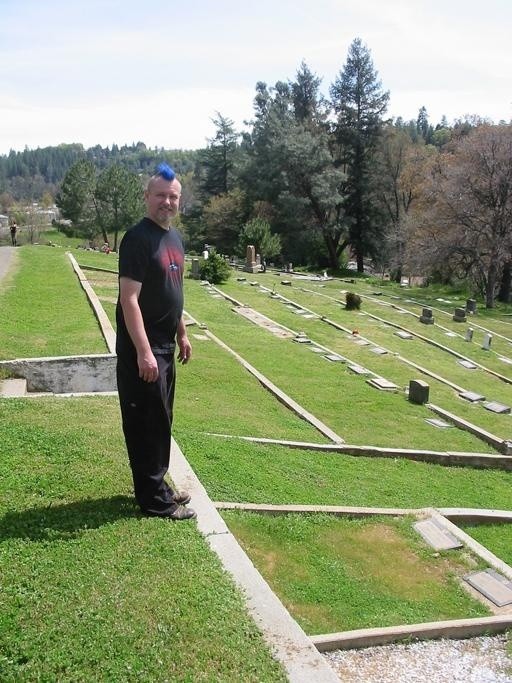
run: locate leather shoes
[140,505,198,521]
[170,490,192,504]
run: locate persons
[114,162,198,520]
[10,220,17,247]
